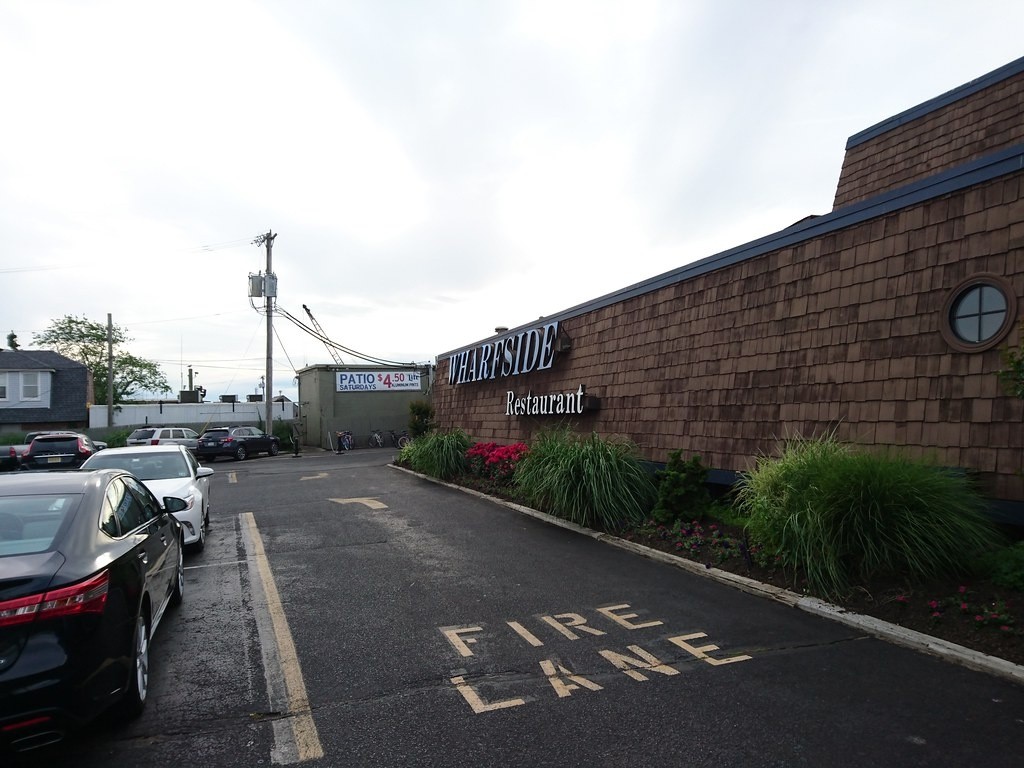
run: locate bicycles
[368,429,386,448]
[335,430,354,450]
[391,429,412,449]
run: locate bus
[198,425,281,461]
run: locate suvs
[126,426,203,457]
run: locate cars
[0,469,181,752]
[47,446,216,552]
[20,435,105,472]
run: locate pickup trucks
[0,430,108,464]
